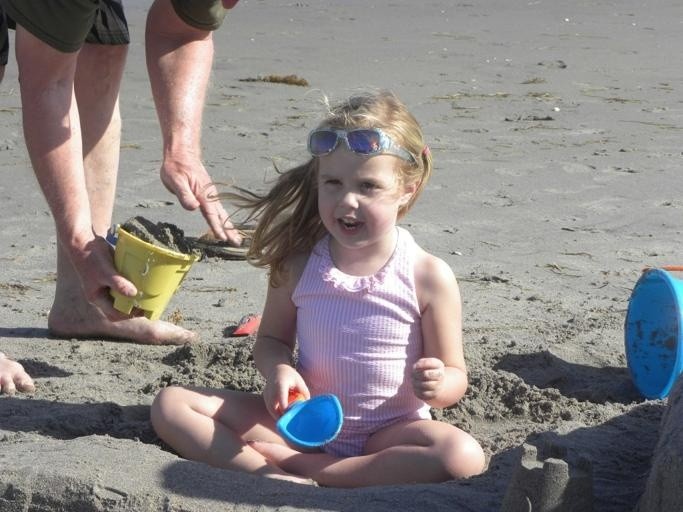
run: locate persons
[0,1,245,397]
[147,88,485,489]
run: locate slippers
[189,229,269,260]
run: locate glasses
[306,126,417,164]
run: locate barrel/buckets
[112,222,201,321]
[625,264,681,399]
